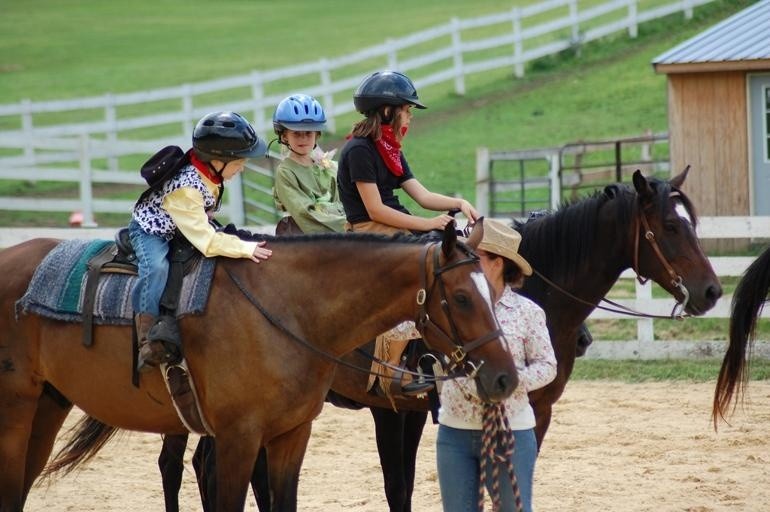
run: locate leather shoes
[401,380,433,396]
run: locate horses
[0,216,521,511]
[45,166,724,511]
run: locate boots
[134,311,176,373]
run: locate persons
[129,111,274,367]
[271,94,348,234]
[336,70,479,236]
[383,218,559,512]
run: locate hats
[454,216,533,276]
[134,145,189,205]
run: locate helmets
[352,70,429,113]
[191,110,267,164]
[272,93,327,135]
